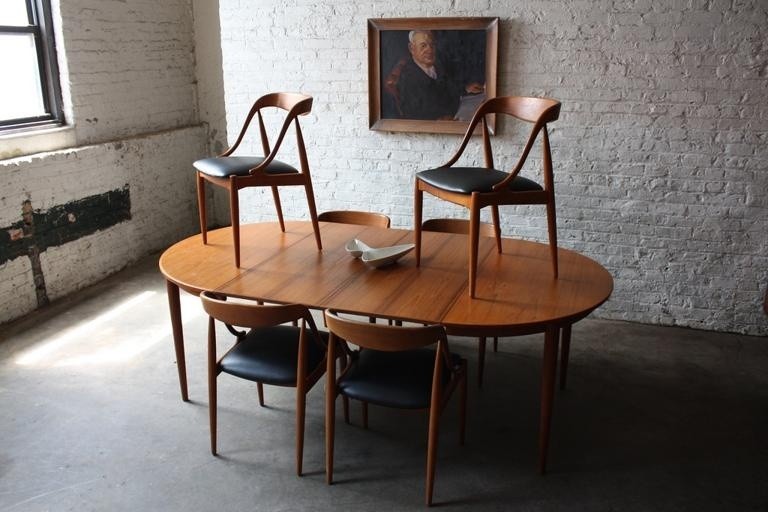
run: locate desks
[158,220,619,477]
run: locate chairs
[194,87,324,269]
[199,290,349,477]
[293,211,393,351]
[325,308,469,506]
[395,219,496,387]
[410,92,566,302]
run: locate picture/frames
[364,16,504,139]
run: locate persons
[397,29,483,121]
